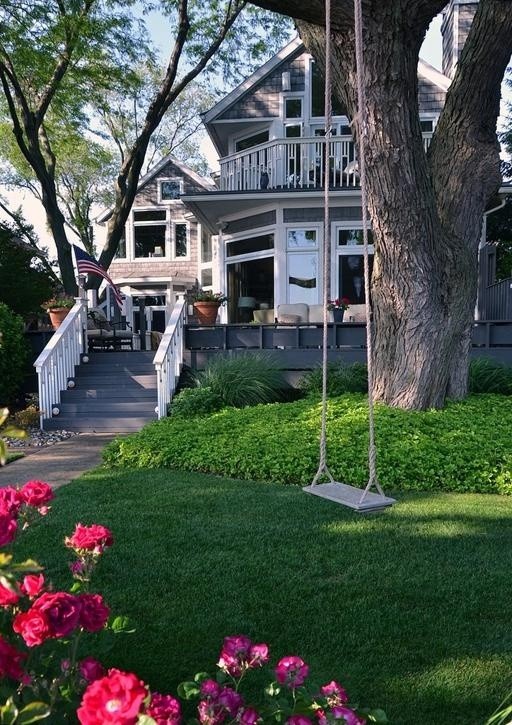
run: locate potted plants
[41,297,76,331]
[188,287,229,325]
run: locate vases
[333,311,344,324]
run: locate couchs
[276,302,309,329]
[309,304,367,323]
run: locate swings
[300,0,397,512]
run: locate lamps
[237,296,257,309]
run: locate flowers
[326,297,351,311]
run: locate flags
[73,245,125,309]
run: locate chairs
[87,310,135,354]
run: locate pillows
[278,313,301,325]
